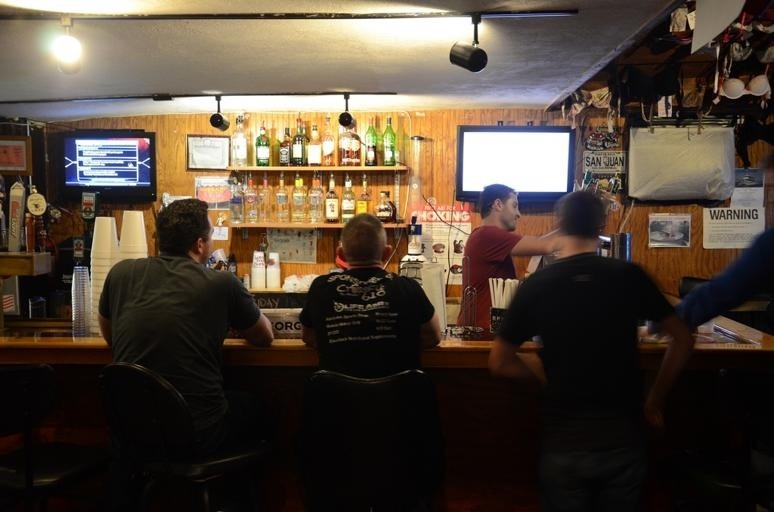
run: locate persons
[456,182,565,330]
[300,212,442,379]
[98,198,274,510]
[637,151,773,512]
[485,188,696,511]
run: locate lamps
[449,15,487,72]
[210,96,229,131]
[339,94,356,129]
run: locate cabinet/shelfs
[226,166,408,294]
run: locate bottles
[326,172,339,223]
[290,119,308,166]
[382,113,396,166]
[231,114,248,167]
[357,173,373,217]
[245,171,258,224]
[341,172,356,224]
[365,115,379,166]
[273,171,289,223]
[255,126,271,167]
[292,173,307,222]
[279,128,290,166]
[229,173,244,223]
[322,113,335,166]
[308,125,321,166]
[337,119,362,166]
[258,171,273,222]
[308,170,324,223]
[376,190,396,223]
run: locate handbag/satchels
[628,121,736,204]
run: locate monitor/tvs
[456,124,576,203]
[62,129,157,204]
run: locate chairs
[0,359,104,510]
[657,365,774,512]
[292,369,438,510]
[101,362,260,510]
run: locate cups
[120,210,149,259]
[252,250,265,288]
[72,266,91,338]
[267,253,281,289]
[90,216,119,333]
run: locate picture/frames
[185,135,231,172]
[0,134,31,177]
